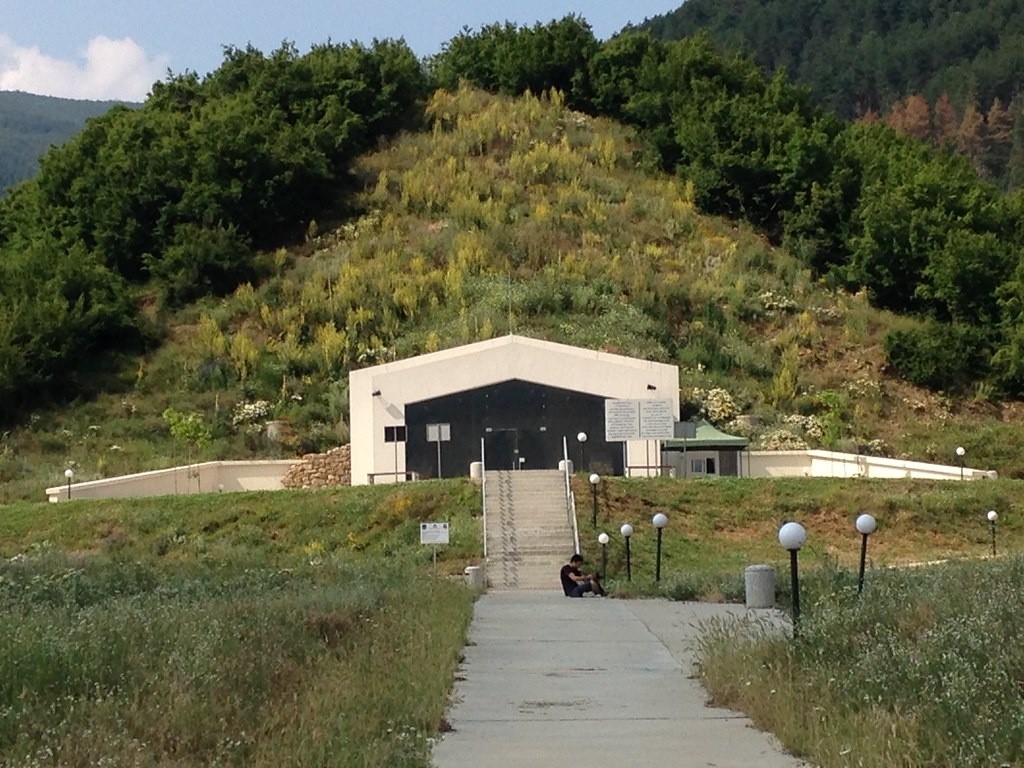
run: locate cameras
[591,572,601,582]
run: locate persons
[559,554,609,598]
